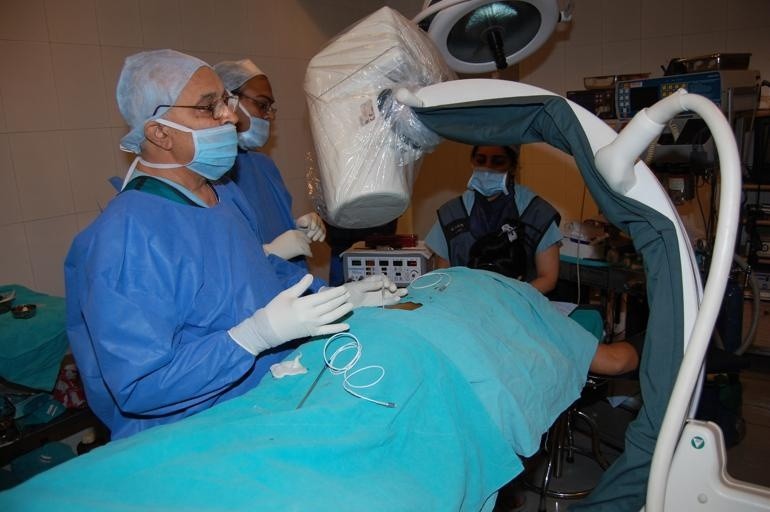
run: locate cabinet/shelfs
[0,280,111,469]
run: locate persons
[211,59,328,275]
[466,233,644,377]
[60,47,411,439]
[422,141,565,295]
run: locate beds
[0,295,613,511]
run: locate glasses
[149,94,239,122]
[232,89,277,117]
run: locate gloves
[321,273,411,309]
[261,229,314,263]
[295,212,328,243]
[225,272,354,358]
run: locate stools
[511,375,610,512]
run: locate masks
[235,115,272,153]
[187,123,238,182]
[465,167,513,197]
